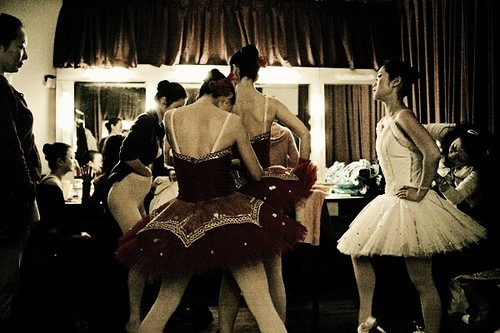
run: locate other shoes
[357,316,377,333]
[125,322,141,333]
[461,314,471,325]
[413,331,425,333]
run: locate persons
[107,44,311,333]
[0,12,42,333]
[336,60,500,333]
[36,143,91,237]
[90,135,125,235]
[74,108,126,174]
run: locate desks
[282,183,416,320]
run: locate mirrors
[56,66,326,189]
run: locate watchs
[438,178,447,186]
